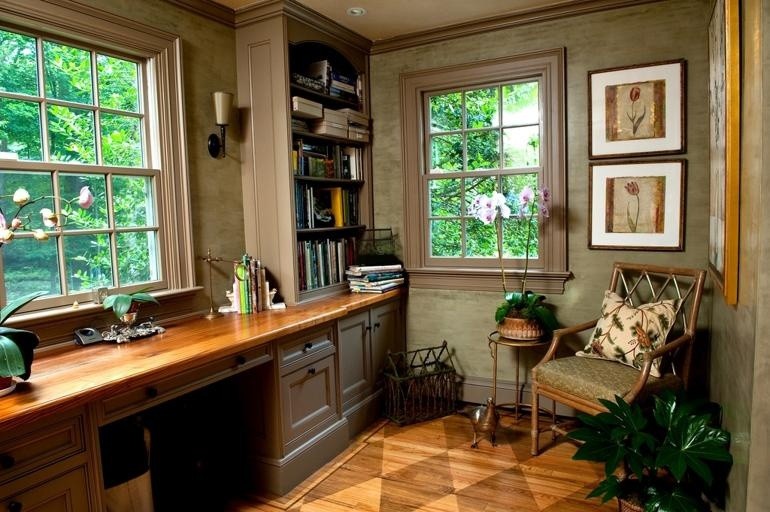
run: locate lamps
[204,88,236,162]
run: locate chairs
[526,259,708,459]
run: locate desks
[486,330,561,445]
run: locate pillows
[573,289,684,381]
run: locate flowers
[0,133,96,242]
[469,183,559,328]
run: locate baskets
[385,341,458,427]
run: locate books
[288,56,372,295]
[343,261,408,296]
[232,253,271,315]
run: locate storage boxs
[291,94,371,145]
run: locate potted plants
[101,286,161,321]
[561,395,732,511]
[495,315,545,342]
[1,288,49,398]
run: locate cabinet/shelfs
[339,287,407,440]
[236,2,376,308]
[2,400,107,511]
[263,311,349,496]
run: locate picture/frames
[585,58,688,160]
[703,0,743,308]
[586,158,688,253]
